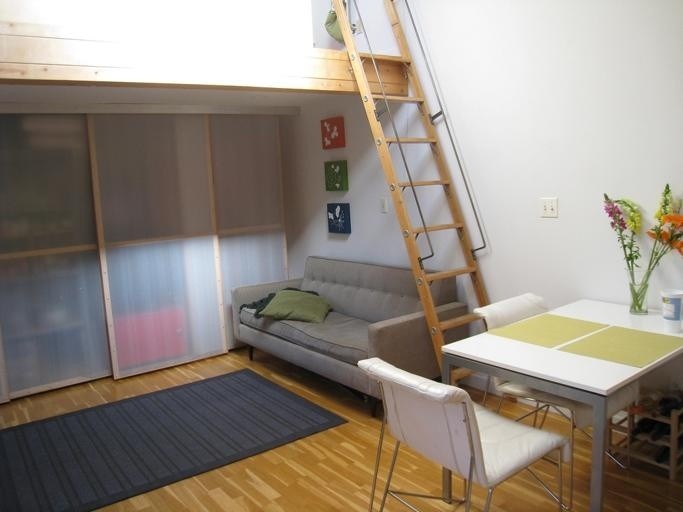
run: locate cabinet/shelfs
[607,401,683,480]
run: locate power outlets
[540,198,557,217]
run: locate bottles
[629,394,681,465]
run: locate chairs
[473,293,631,511]
[357,357,570,512]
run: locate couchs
[230,255,468,417]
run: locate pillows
[258,289,331,324]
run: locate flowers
[603,184,683,312]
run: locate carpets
[0,367,349,512]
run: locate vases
[625,268,649,315]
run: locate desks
[441,299,683,512]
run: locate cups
[659,288,682,334]
